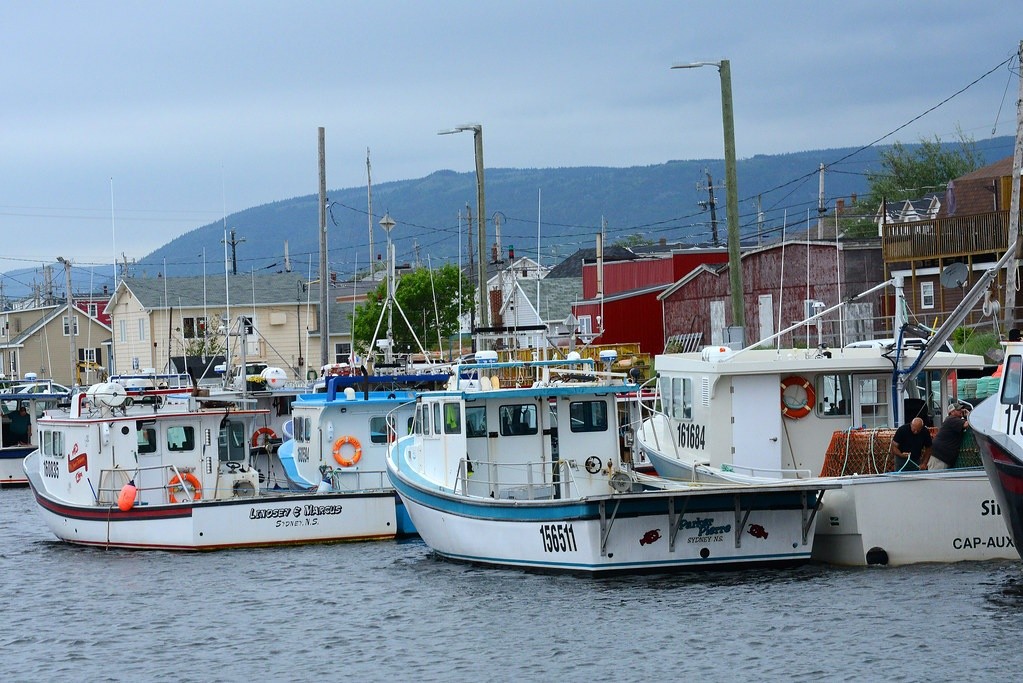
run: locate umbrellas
[946,178,957,217]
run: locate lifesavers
[781,376,817,420]
[252,427,278,449]
[332,435,361,467]
[166,472,203,504]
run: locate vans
[841,336,954,385]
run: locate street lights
[436,124,489,350]
[219,238,246,274]
[670,60,744,328]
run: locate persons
[891,418,932,472]
[926,403,969,471]
[1,407,32,447]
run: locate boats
[0,143,1023,576]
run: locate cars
[452,353,477,364]
[66,385,92,406]
[231,361,270,390]
[3,383,72,411]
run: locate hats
[947,403,963,414]
